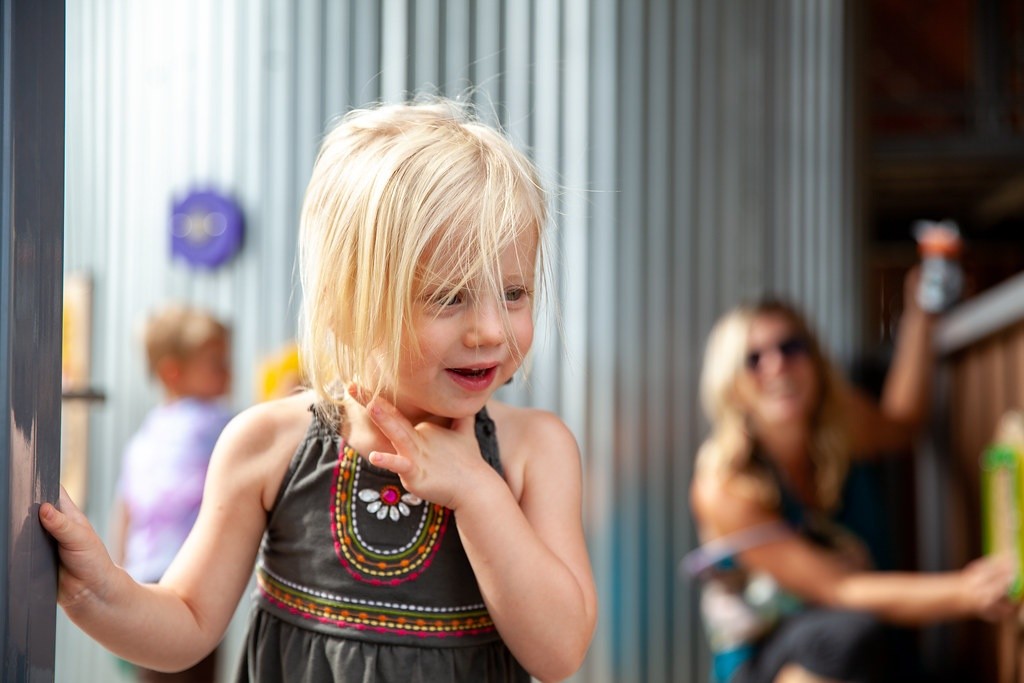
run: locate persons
[39,104,594,683]
[682,268,1014,683]
[115,309,233,683]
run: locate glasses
[747,338,804,369]
